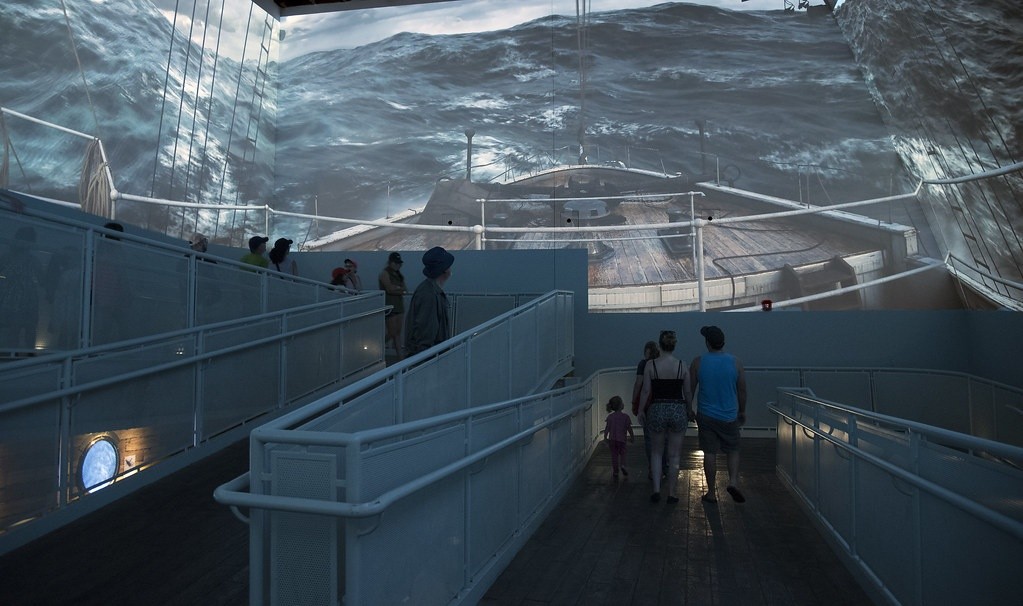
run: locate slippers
[727,486,746,503]
[701,494,718,504]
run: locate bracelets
[632,402,635,404]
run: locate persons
[631,341,669,479]
[637,330,694,504]
[327,258,361,296]
[690,326,746,504]
[269,238,293,281]
[405,246,455,368]
[101,223,123,241]
[605,396,634,478]
[185,233,216,264]
[240,236,269,273]
[379,252,408,361]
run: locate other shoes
[621,467,627,474]
[652,492,660,502]
[613,472,618,476]
[648,473,653,480]
[667,495,679,504]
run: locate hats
[422,246,455,278]
[249,236,269,247]
[390,252,403,263]
[701,326,724,345]
[275,238,293,248]
[345,259,358,268]
